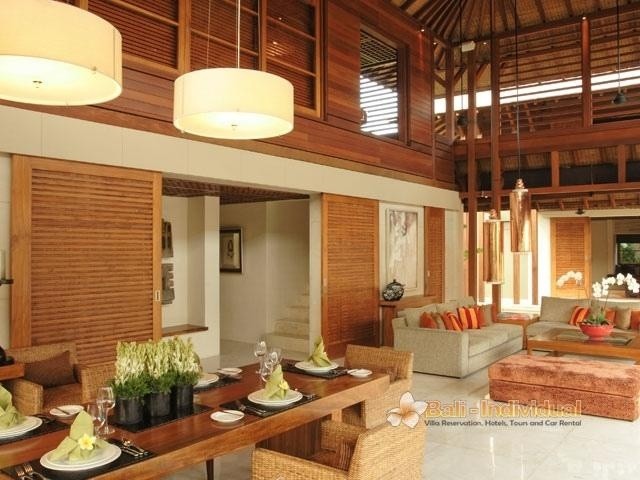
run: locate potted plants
[109,339,148,426]
[143,339,178,418]
[170,336,204,416]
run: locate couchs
[391,296,524,379]
[525,296,640,352]
[488,354,640,422]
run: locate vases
[578,320,616,341]
[382,286,395,301]
[387,278,404,300]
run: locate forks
[15,466,31,480]
[23,463,49,480]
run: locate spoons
[122,437,145,452]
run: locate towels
[307,336,332,368]
[262,365,289,399]
[0,384,26,430]
[49,411,106,463]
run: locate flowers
[555,270,640,323]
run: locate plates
[200,373,219,385]
[248,389,303,407]
[40,444,122,471]
[50,405,83,416]
[210,410,245,423]
[220,368,242,375]
[347,369,372,378]
[0,416,42,438]
[295,362,338,373]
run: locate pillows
[463,305,487,327]
[614,307,631,330]
[21,349,76,389]
[431,311,446,329]
[630,308,640,330]
[568,306,591,327]
[456,306,480,329]
[440,313,454,330]
[605,307,615,322]
[446,310,463,332]
[422,311,437,330]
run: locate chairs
[342,343,414,429]
[250,412,427,480]
[80,360,116,403]
[3,341,82,416]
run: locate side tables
[496,311,540,350]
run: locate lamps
[172,0,295,140]
[509,0,531,254]
[612,1,628,104]
[482,0,505,285]
[0,0,123,107]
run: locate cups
[97,387,116,436]
[87,404,105,440]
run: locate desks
[379,293,437,348]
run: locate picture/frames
[219,228,243,273]
[385,208,419,293]
